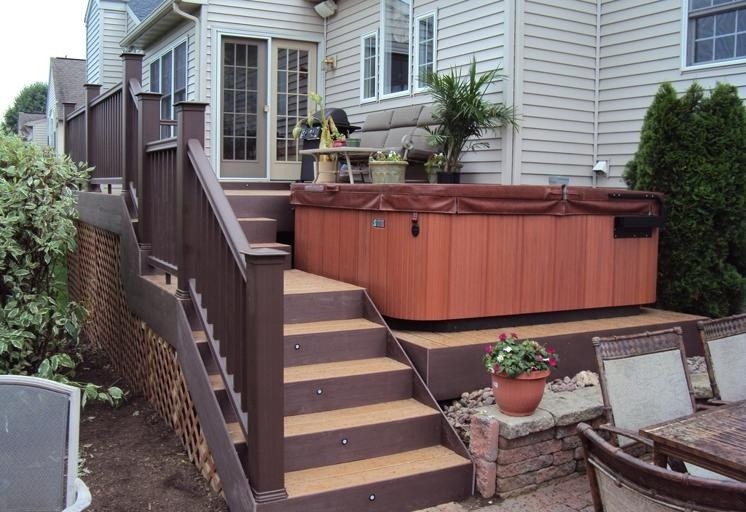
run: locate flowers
[484,332,559,376]
[292,91,347,160]
[424,152,447,170]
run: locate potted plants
[414,54,520,184]
[368,150,408,183]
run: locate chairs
[593,327,701,453]
[0,376,94,511]
[698,312,746,403]
[576,421,746,512]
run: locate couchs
[347,105,454,181]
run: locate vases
[426,167,444,183]
[490,365,550,417]
[315,160,337,184]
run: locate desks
[637,396,745,483]
